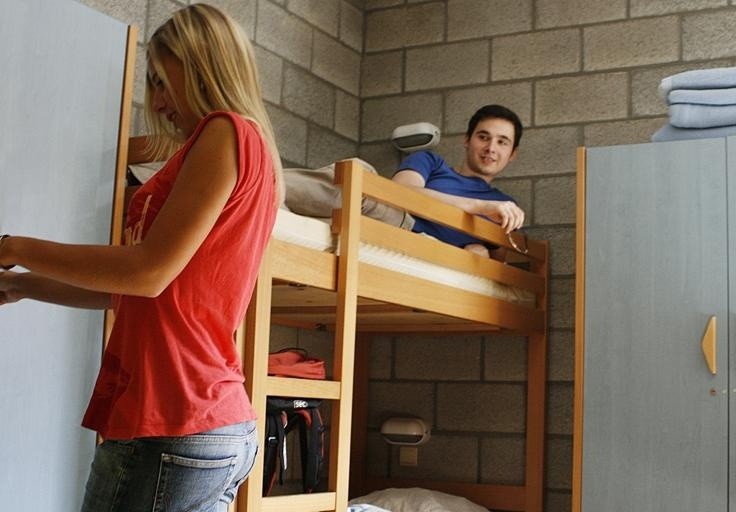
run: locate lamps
[391,122,441,153]
[380,417,431,446]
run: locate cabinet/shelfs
[570,136,735,512]
[0,0,136,512]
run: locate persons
[282,104,527,267]
[1,1,286,511]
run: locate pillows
[348,487,490,512]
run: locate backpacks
[263,347,326,496]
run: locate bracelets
[0,233,17,273]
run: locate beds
[122,134,551,512]
